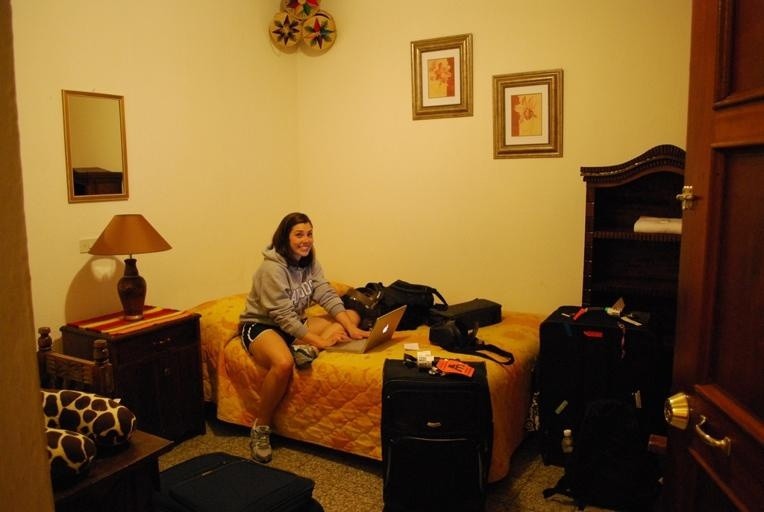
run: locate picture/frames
[489,68,565,162]
[409,32,472,121]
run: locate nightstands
[57,304,205,453]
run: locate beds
[189,279,549,485]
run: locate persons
[239,211,372,464]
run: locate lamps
[88,213,173,321]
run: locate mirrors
[60,87,130,203]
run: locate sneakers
[291,343,319,369]
[250,417,272,463]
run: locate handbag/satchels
[428,311,480,353]
[339,279,435,331]
[427,298,502,329]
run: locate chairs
[32,323,115,398]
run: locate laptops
[325,304,407,354]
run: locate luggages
[534,306,661,467]
[382,353,493,512]
[160,451,325,512]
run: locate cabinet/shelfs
[41,428,174,511]
[578,144,684,316]
[0,0,60,511]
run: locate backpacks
[543,397,660,512]
